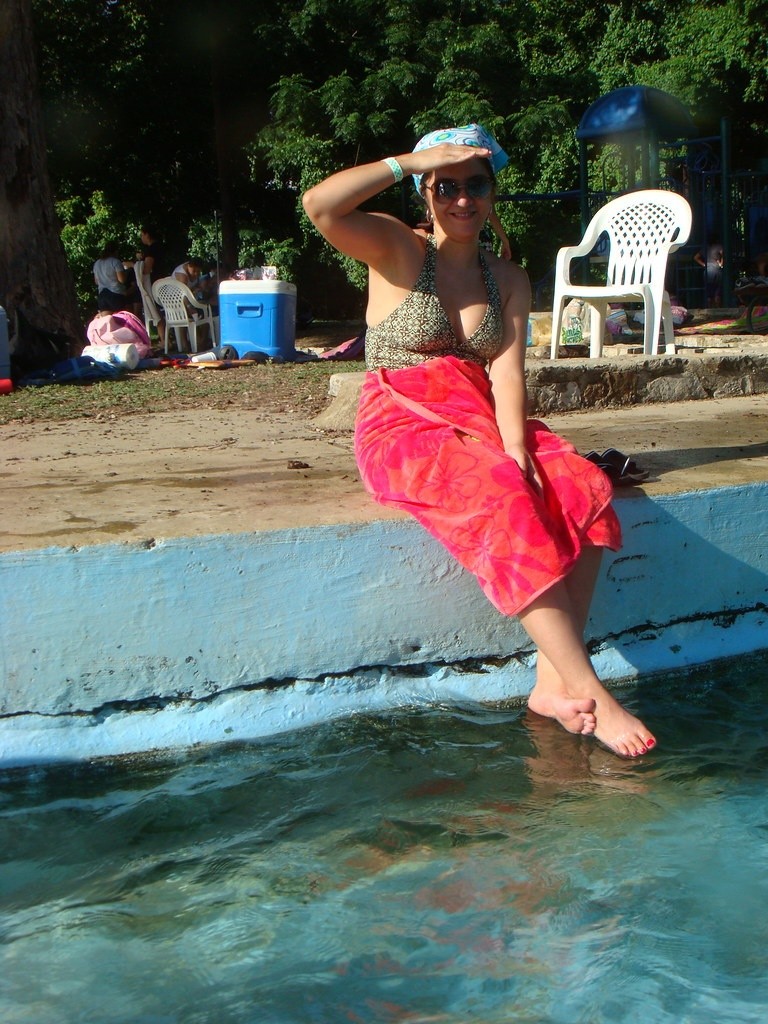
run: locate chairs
[550,189,692,361]
[151,278,216,354]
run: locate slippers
[581,450,632,483]
[599,447,650,481]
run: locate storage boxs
[218,280,297,362]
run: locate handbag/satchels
[86,314,149,359]
[112,310,151,350]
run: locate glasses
[420,174,496,200]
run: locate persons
[93,224,222,353]
[693,233,724,309]
[302,123,656,759]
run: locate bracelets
[381,157,404,183]
